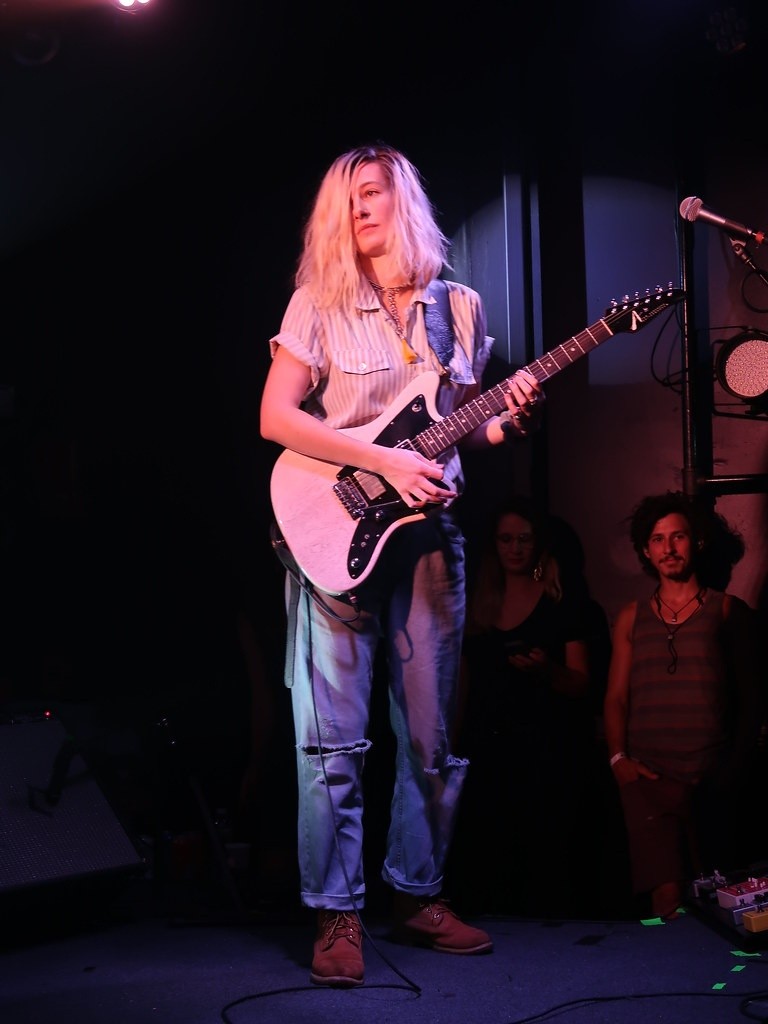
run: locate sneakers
[399,894,493,953]
[310,910,364,985]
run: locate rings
[515,415,520,420]
[529,395,538,405]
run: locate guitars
[267,281,689,601]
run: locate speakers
[0,719,144,952]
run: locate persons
[603,493,753,921]
[438,496,630,917]
[258,145,547,987]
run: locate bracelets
[611,752,626,767]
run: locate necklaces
[653,582,708,674]
[368,274,418,365]
[659,592,697,622]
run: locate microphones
[680,196,768,247]
